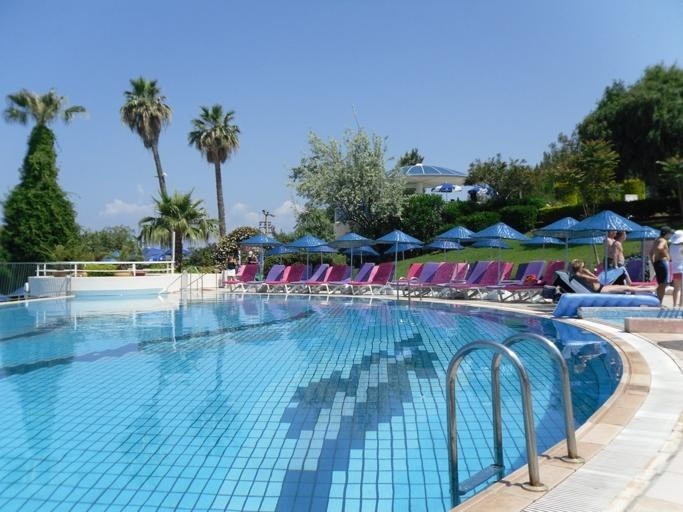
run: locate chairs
[224,294,540,329]
[220,258,673,308]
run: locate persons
[650,227,674,306]
[667,230,683,310]
